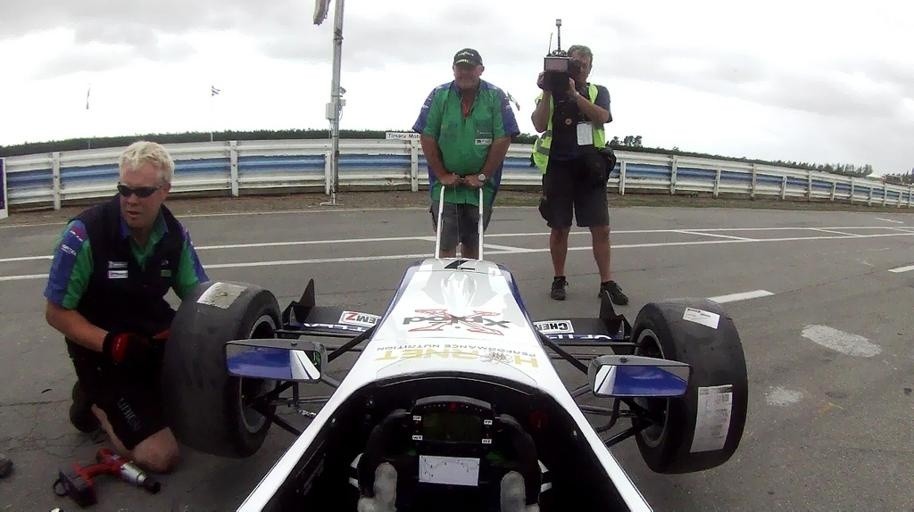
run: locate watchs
[477,171,489,184]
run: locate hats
[454,49,483,66]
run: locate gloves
[500,471,540,512]
[357,462,398,512]
[102,329,169,366]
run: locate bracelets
[570,90,580,100]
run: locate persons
[412,48,520,263]
[42,140,212,474]
[530,44,631,305]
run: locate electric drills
[60,450,162,509]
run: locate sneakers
[598,281,628,305]
[551,276,568,300]
[70,380,98,432]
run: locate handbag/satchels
[582,147,617,189]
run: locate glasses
[117,181,165,197]
